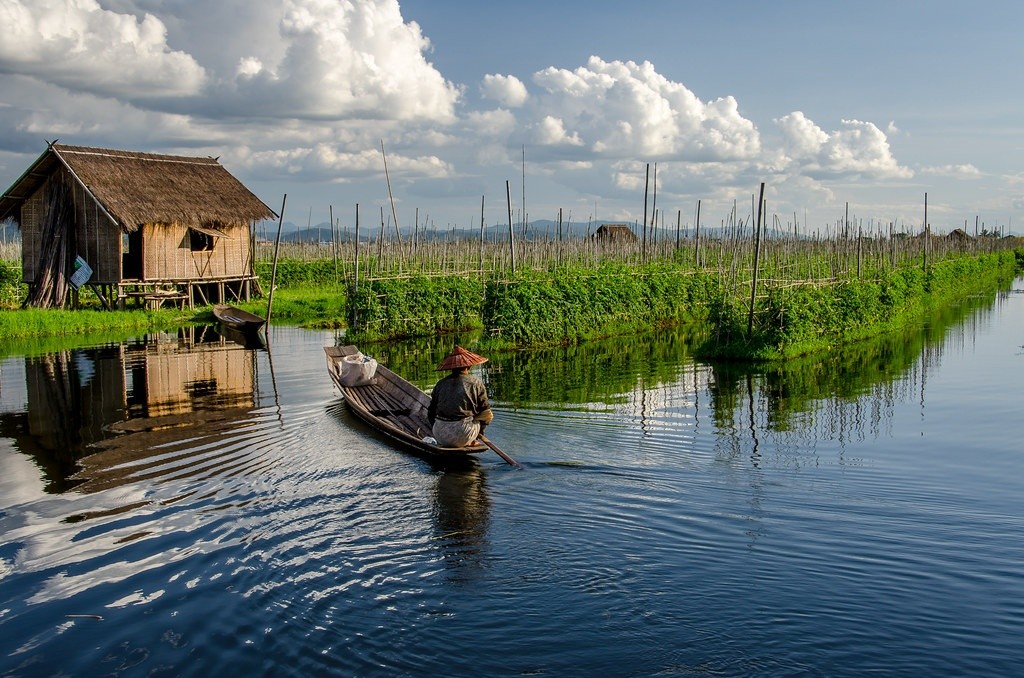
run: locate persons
[428,346,494,447]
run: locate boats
[212,305,267,331]
[323,344,489,454]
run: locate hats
[436,345,488,370]
